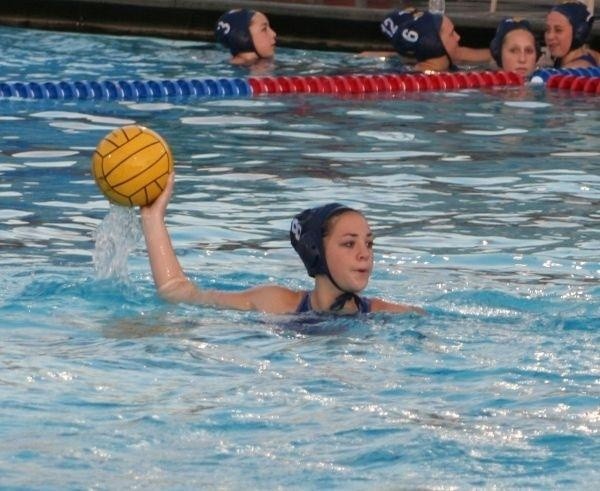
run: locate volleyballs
[92,125,174,207]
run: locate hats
[382,8,446,63]
[216,8,255,54]
[553,2,593,50]
[490,17,538,68]
[289,203,352,277]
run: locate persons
[137,172,433,341]
[350,0,600,80]
[215,8,278,72]
